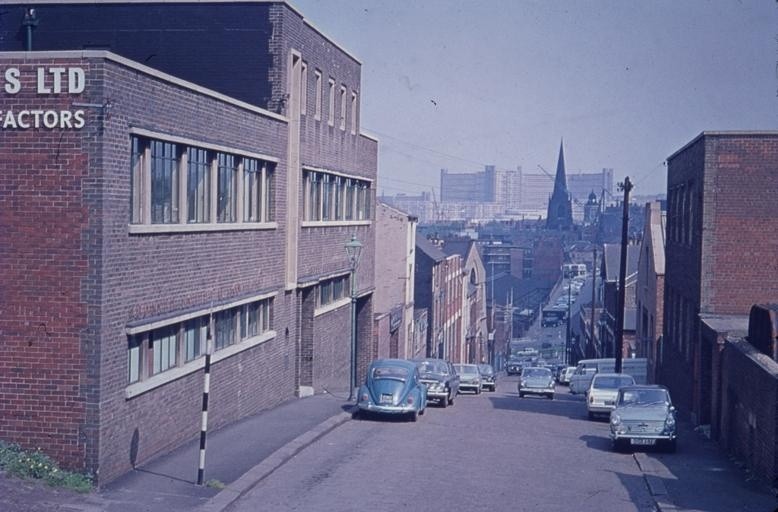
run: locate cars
[506,348,679,452]
[357,358,496,422]
[541,277,585,327]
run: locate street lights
[344,235,365,401]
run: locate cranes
[538,164,604,241]
